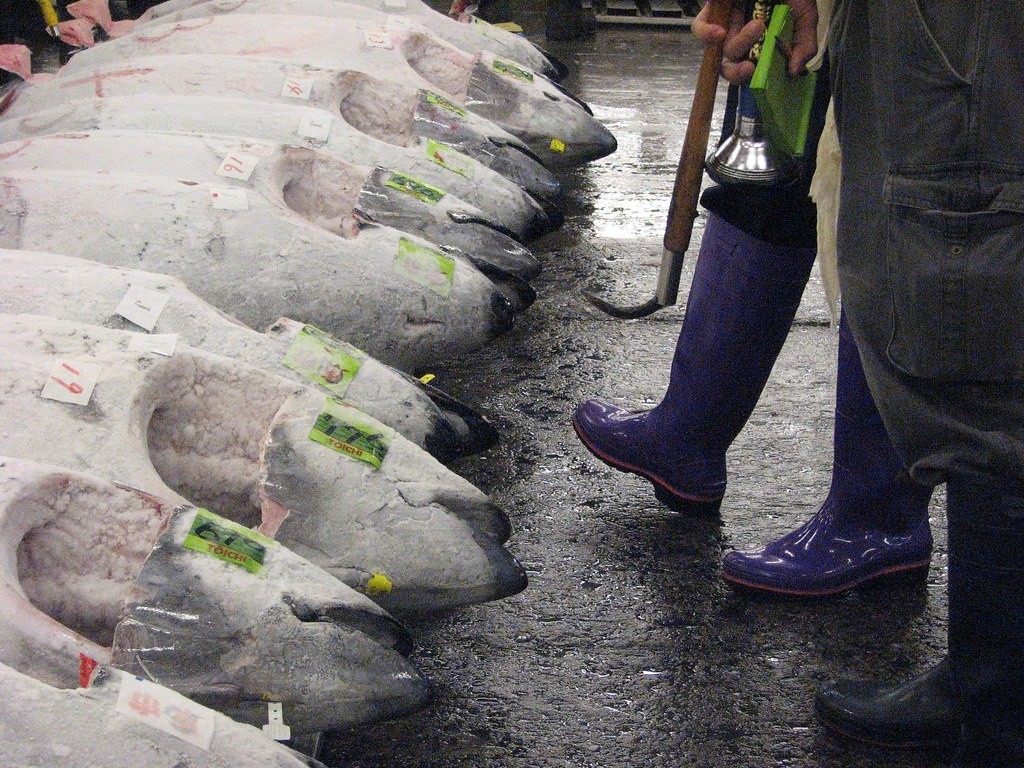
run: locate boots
[573,208,815,514]
[721,300,931,594]
[814,477,1024,749]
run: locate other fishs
[1,0,618,768]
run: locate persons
[691,0,1024,748]
[571,0,933,597]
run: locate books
[749,5,817,155]
